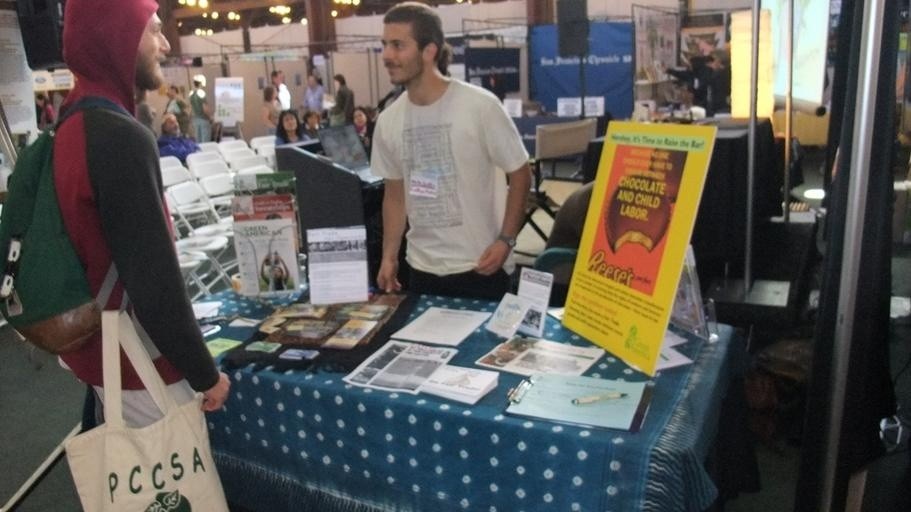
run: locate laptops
[315,124,383,185]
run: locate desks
[186,291,737,512]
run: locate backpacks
[0,96,132,353]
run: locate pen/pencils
[572,393,627,405]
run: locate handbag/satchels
[64,395,233,512]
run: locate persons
[264,66,407,163]
[667,56,726,118]
[543,178,599,306]
[50,1,231,424]
[135,74,212,160]
[437,43,453,77]
[489,74,505,102]
[34,90,70,131]
[371,3,534,292]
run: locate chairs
[524,178,585,211]
[154,134,278,301]
[705,212,823,344]
[524,119,596,182]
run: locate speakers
[15,0,68,72]
[583,128,783,292]
[691,117,803,234]
[555,1,588,59]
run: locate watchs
[498,234,517,249]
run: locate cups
[688,297,720,345]
